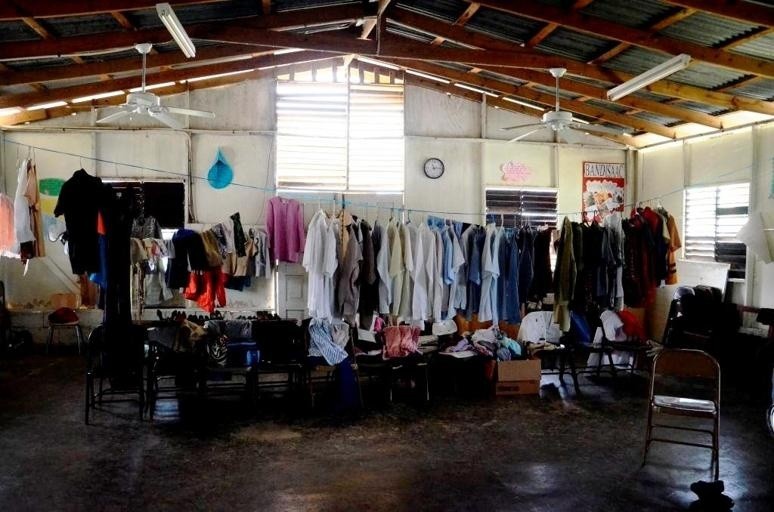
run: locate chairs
[46,293,82,352]
[642,348,720,479]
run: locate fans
[499,68,610,144]
[66,44,215,131]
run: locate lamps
[154,3,196,60]
[606,54,691,102]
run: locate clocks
[423,158,444,179]
